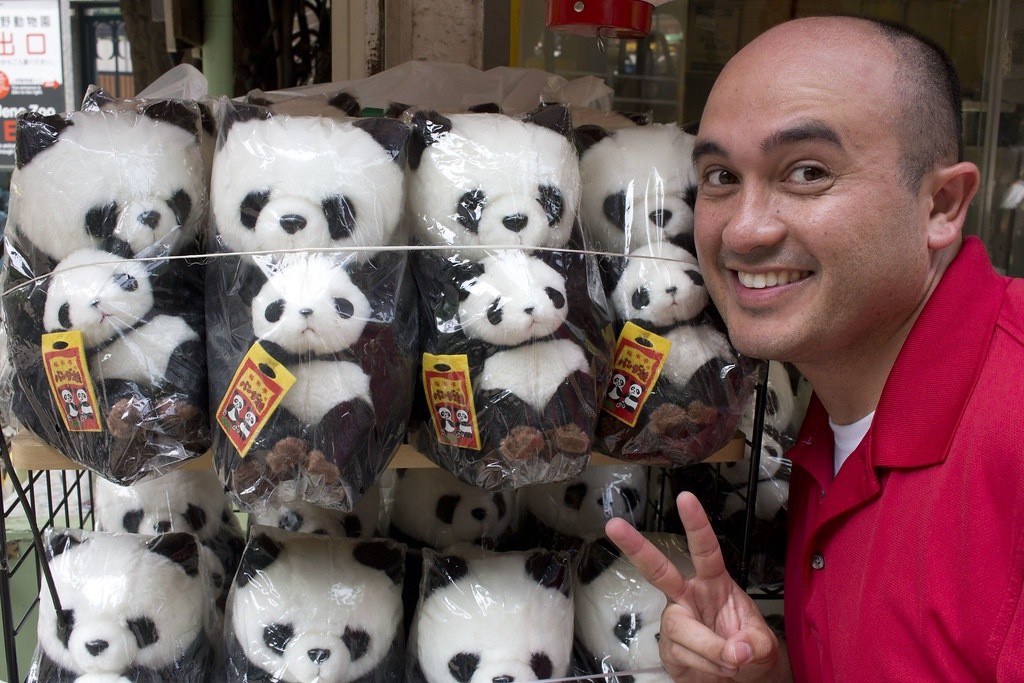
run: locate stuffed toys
[1,85,811,683]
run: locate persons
[603,13,1024,680]
